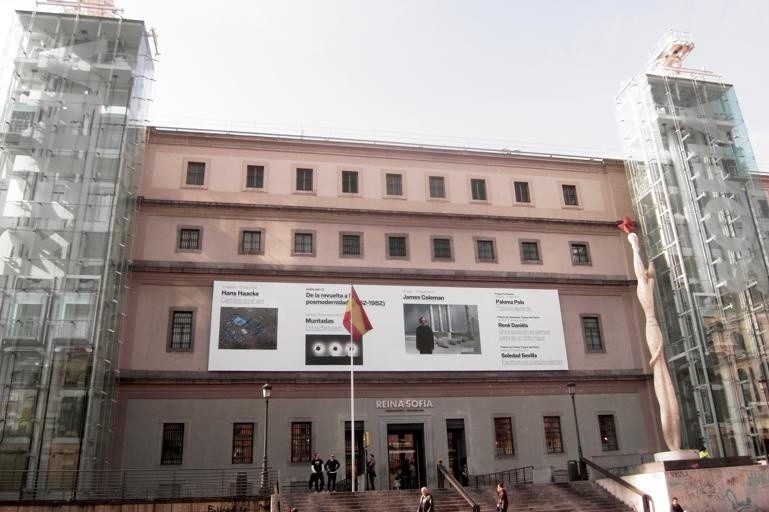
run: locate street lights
[260,383,272,494]
[566,381,588,480]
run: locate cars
[0,338,95,388]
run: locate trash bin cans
[567,460,579,481]
[236,472,247,495]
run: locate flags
[342,282,374,343]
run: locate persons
[698,446,712,459]
[367,452,376,491]
[417,486,435,512]
[324,453,340,493]
[496,482,508,512]
[311,451,325,493]
[415,315,435,354]
[670,496,683,511]
[392,477,401,492]
